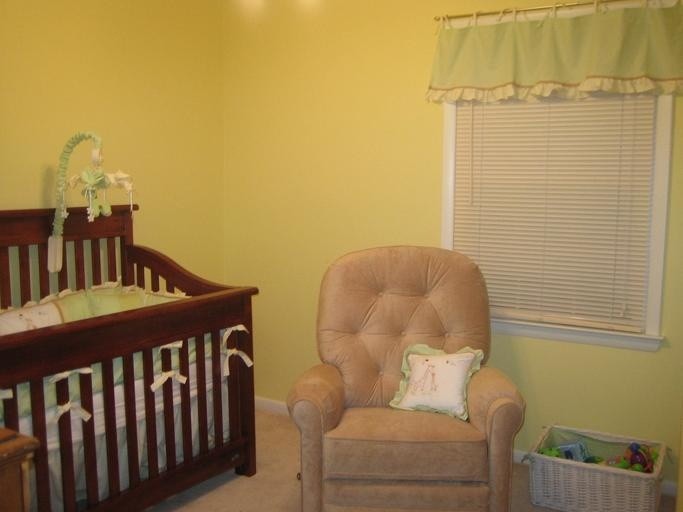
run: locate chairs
[285,244,530,512]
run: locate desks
[0,425,42,512]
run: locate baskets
[528,424,667,512]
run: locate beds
[0,205,261,512]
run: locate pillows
[388,341,484,422]
